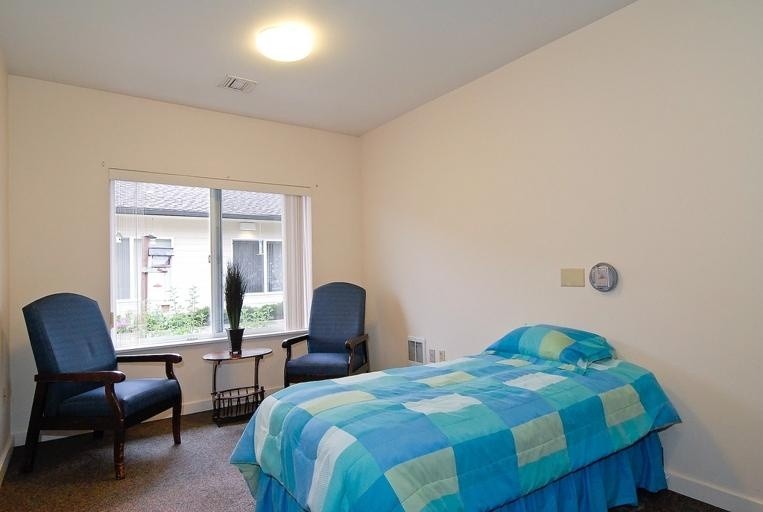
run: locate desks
[201,346,272,427]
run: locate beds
[228,357,682,512]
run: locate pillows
[481,324,618,376]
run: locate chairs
[281,282,372,389]
[20,292,182,480]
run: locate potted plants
[224,260,249,354]
